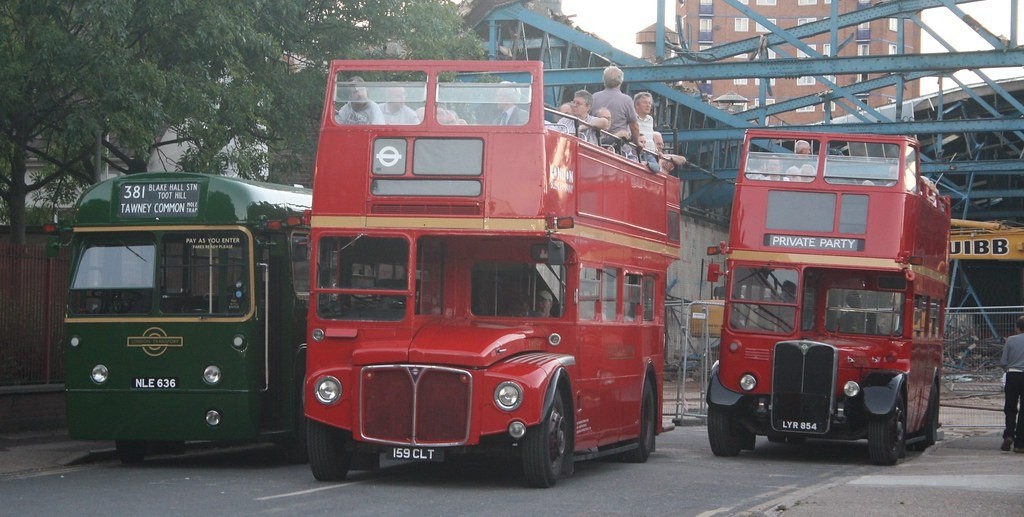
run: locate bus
[41,169,425,467]
[698,130,957,467]
[287,54,685,492]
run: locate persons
[999,316,1024,452]
[335,75,422,125]
[862,166,945,210]
[501,288,555,317]
[836,291,898,334]
[436,80,530,126]
[746,139,816,181]
[544,65,663,172]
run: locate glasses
[599,116,611,121]
[571,101,587,106]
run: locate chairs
[126,293,217,316]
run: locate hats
[537,290,553,302]
[346,76,365,86]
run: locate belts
[1006,368,1023,372]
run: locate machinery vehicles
[688,215,1024,338]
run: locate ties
[502,112,507,125]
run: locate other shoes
[1001,436,1013,451]
[1014,448,1024,453]
